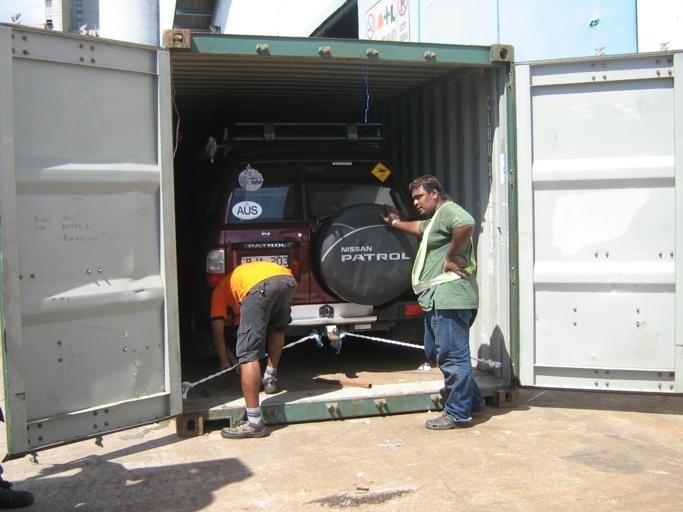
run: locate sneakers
[262,376,279,394]
[426,413,470,430]
[440,396,485,413]
[221,420,266,438]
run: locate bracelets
[391,219,399,227]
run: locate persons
[208,261,296,440]
[379,172,486,432]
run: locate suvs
[200,121,425,365]
